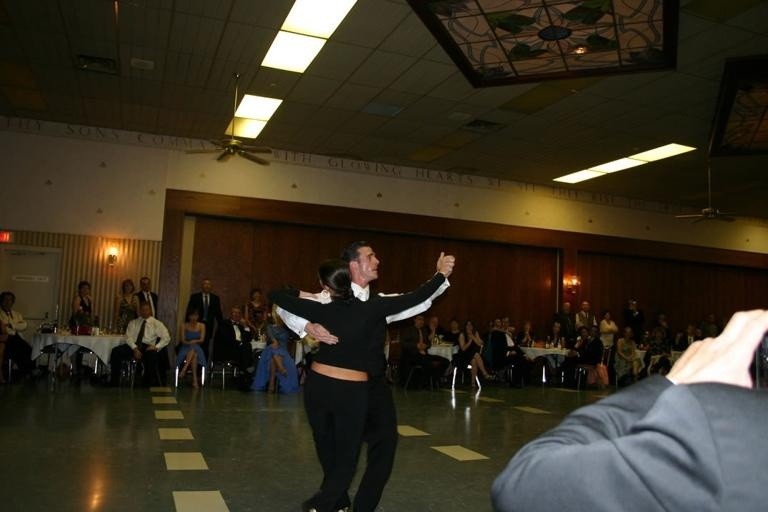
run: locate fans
[675,126,737,224]
[184,72,273,166]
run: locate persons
[598,309,618,367]
[570,326,591,351]
[616,326,639,377]
[423,316,446,347]
[493,318,516,337]
[111,301,171,388]
[72,281,94,330]
[177,309,207,388]
[642,329,673,379]
[626,296,645,347]
[136,277,158,318]
[575,301,598,333]
[675,325,701,351]
[186,280,225,362]
[558,302,576,337]
[218,306,258,392]
[254,303,298,392]
[114,278,140,334]
[492,317,536,389]
[245,288,267,331]
[400,312,446,390]
[443,320,462,346]
[458,319,496,388]
[519,321,536,347]
[0,291,40,378]
[489,309,768,511]
[545,321,566,375]
[571,325,605,387]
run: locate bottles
[545,336,550,347]
[558,339,561,350]
[434,328,439,348]
[550,338,554,347]
[259,329,261,342]
[532,339,535,347]
[528,339,531,347]
[54,320,58,333]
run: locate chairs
[577,347,612,392]
[505,364,524,387]
[174,341,214,387]
[8,356,18,385]
[391,340,438,396]
[616,373,622,388]
[120,337,166,387]
[451,344,484,383]
[264,369,280,392]
[209,343,237,390]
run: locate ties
[417,325,424,344]
[146,290,152,316]
[205,295,209,322]
[136,319,147,347]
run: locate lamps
[107,248,118,267]
[553,130,699,187]
[567,271,582,294]
[225,83,283,141]
[260,0,362,73]
